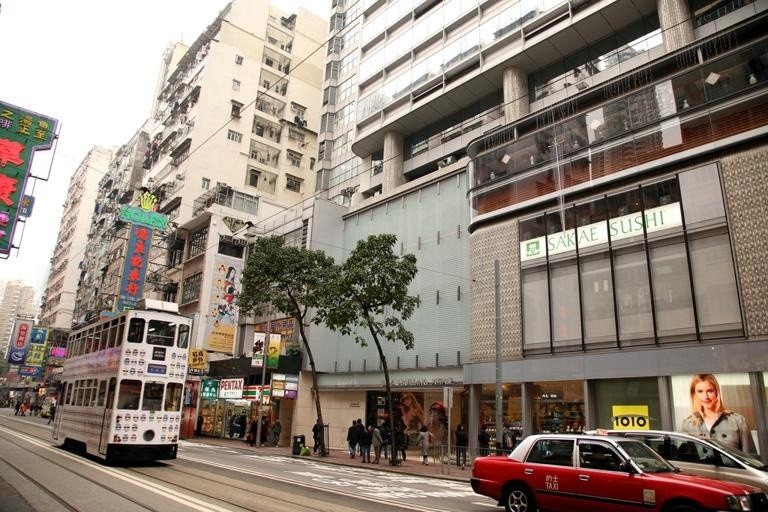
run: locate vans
[41,396,55,418]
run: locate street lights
[244,233,274,448]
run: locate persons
[346,417,406,465]
[217,267,239,325]
[502,422,516,455]
[237,411,246,438]
[571,422,581,434]
[183,381,195,407]
[121,398,136,409]
[14,395,56,424]
[455,424,468,467]
[416,425,430,465]
[680,372,758,468]
[144,386,164,400]
[247,415,258,447]
[479,428,490,456]
[195,412,203,437]
[399,391,425,434]
[271,418,281,448]
[312,419,328,454]
[425,402,448,446]
[228,413,236,440]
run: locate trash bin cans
[292,435,305,455]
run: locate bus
[50,298,194,463]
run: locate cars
[470,428,768,511]
